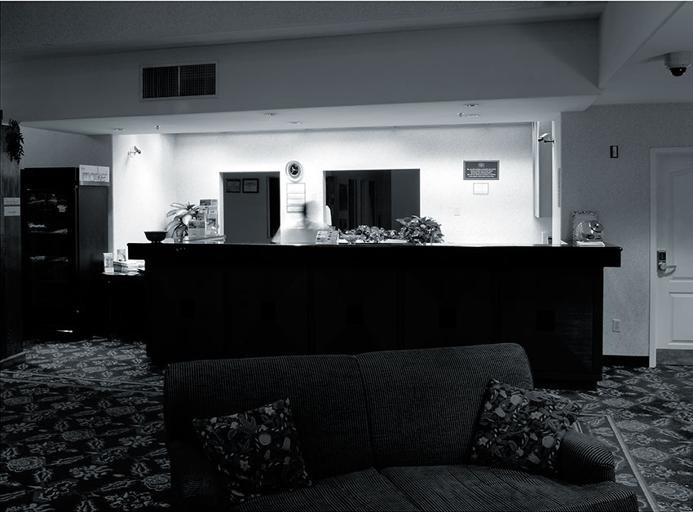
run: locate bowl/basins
[144,230,168,244]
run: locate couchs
[162,343,639,511]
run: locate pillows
[465,376,583,473]
[190,395,312,506]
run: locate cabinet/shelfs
[19,165,112,338]
